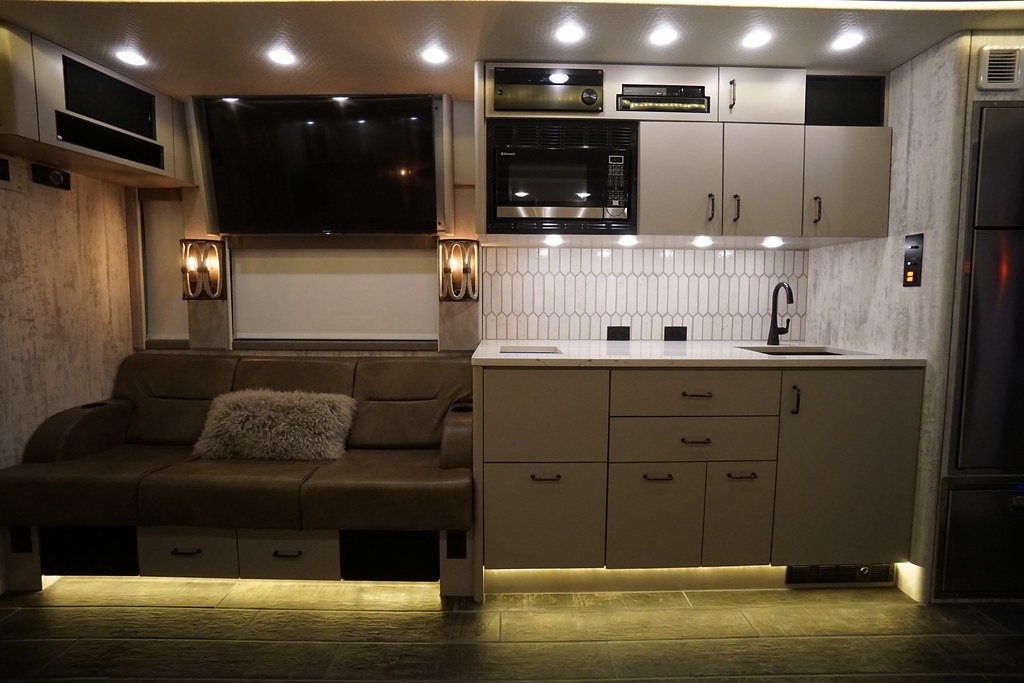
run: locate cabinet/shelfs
[772,368,927,566]
[607,369,783,568]
[802,125,893,240]
[473,366,610,572]
[637,120,805,237]
[719,66,807,123]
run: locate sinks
[737,346,880,355]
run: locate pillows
[189,386,358,463]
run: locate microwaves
[495,143,629,222]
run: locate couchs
[0,352,472,602]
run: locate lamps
[439,238,479,304]
[179,238,227,301]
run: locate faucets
[767,281,794,345]
